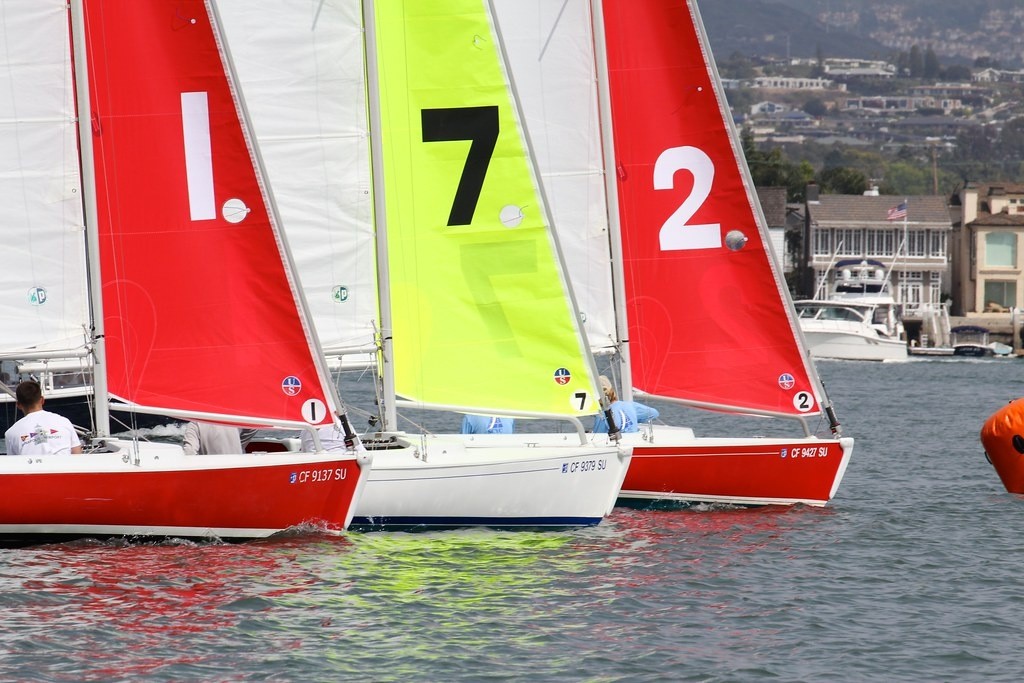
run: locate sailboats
[486,0,859,515]
[211,0,637,531]
[0,0,375,541]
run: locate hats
[599,375,612,393]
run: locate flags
[886,204,907,221]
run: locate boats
[791,239,908,364]
[948,325,1013,357]
[906,338,956,357]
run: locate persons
[588,374,658,433]
[461,413,515,434]
[300,415,363,453]
[181,418,259,454]
[5,381,82,454]
